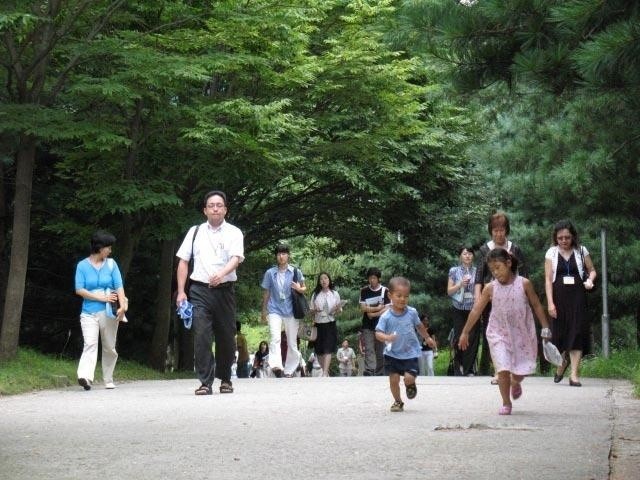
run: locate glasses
[205,202,225,209]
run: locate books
[105,287,129,323]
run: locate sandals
[194,384,212,396]
[219,380,234,394]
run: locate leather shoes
[568,376,582,387]
[554,358,570,383]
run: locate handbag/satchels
[290,267,311,319]
[184,224,201,301]
[578,245,598,295]
[297,308,318,342]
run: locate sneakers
[403,379,417,399]
[389,398,405,412]
[105,382,116,390]
[78,377,93,392]
[511,383,522,400]
[498,402,512,417]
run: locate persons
[175,189,248,395]
[544,219,597,386]
[232,315,481,378]
[309,271,344,377]
[259,243,308,378]
[73,230,127,392]
[473,213,528,385]
[373,274,435,411]
[457,247,553,415]
[446,248,479,376]
[359,268,393,375]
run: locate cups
[464,275,472,285]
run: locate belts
[191,280,233,290]
